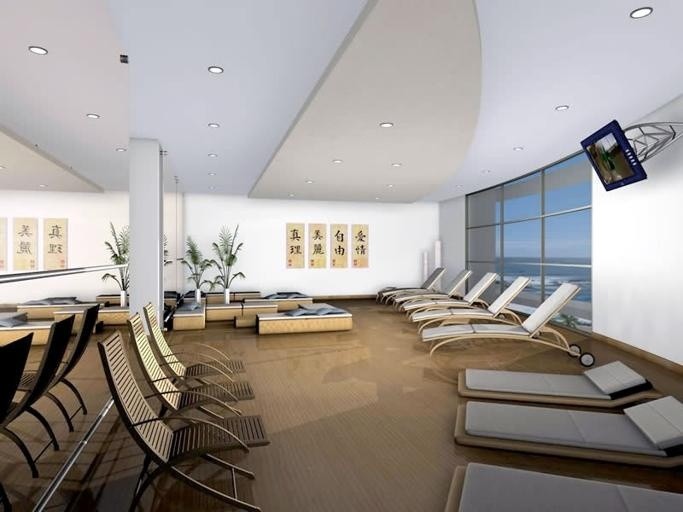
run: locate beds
[443,360,682,512]
[17,294,131,326]
[165,291,354,335]
[96,295,129,305]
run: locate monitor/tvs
[580,119,647,191]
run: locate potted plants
[181,221,246,305]
[99,218,134,308]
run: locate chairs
[378,268,594,370]
[1,305,271,512]
